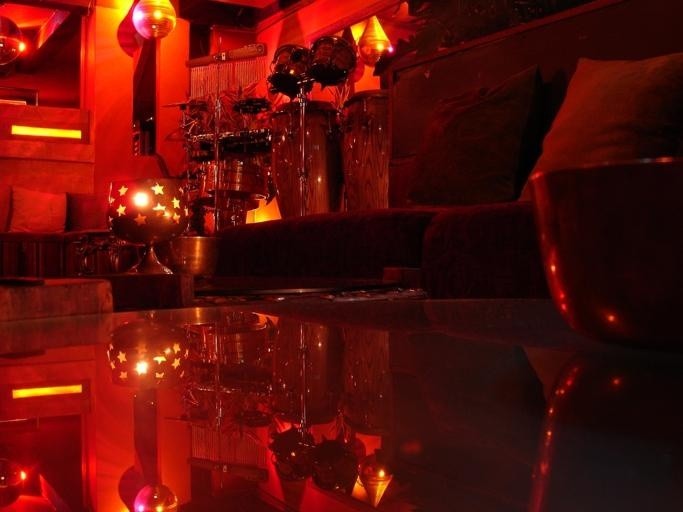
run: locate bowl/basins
[529,155,683,351]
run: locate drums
[217,333,271,369]
[312,440,358,490]
[337,90,389,212]
[240,371,273,394]
[270,100,338,218]
[267,43,314,99]
[270,315,337,425]
[205,159,263,200]
[216,367,240,393]
[246,130,271,153]
[190,134,214,162]
[218,132,245,159]
[197,166,261,211]
[341,320,398,437]
[305,35,356,86]
[269,429,319,478]
[203,333,219,365]
[193,367,216,391]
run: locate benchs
[215,205,547,297]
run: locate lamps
[106,318,189,392]
[0,15,21,66]
[106,180,191,276]
[132,0,177,39]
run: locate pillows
[9,187,67,235]
[514,53,683,207]
[411,65,543,205]
[66,192,107,231]
